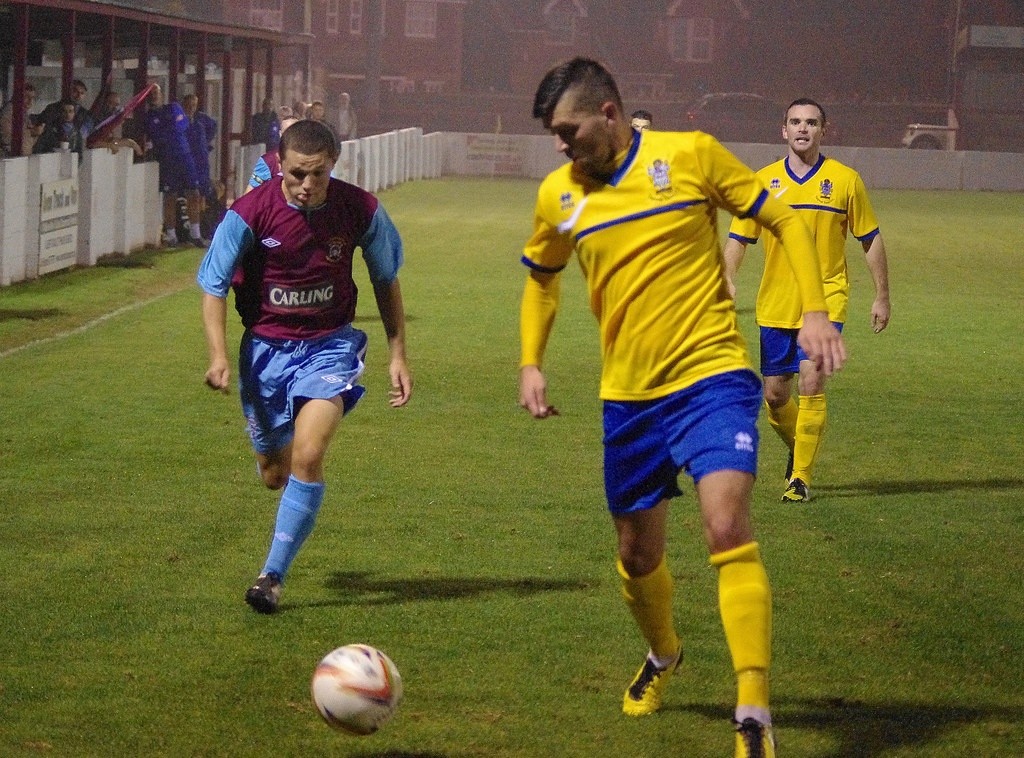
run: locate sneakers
[781,478,809,503]
[784,450,795,488]
[622,638,684,716]
[245,572,283,615]
[731,716,775,758]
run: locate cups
[60,142,69,150]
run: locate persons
[630,110,652,132]
[0,80,222,246]
[245,116,299,194]
[519,58,849,758]
[241,97,278,146]
[724,98,890,502]
[196,121,412,607]
[266,94,358,153]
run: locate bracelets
[115,139,120,145]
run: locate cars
[686,93,784,130]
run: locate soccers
[310,642,403,738]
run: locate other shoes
[168,233,178,245]
[188,234,211,248]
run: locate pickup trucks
[903,124,948,150]
